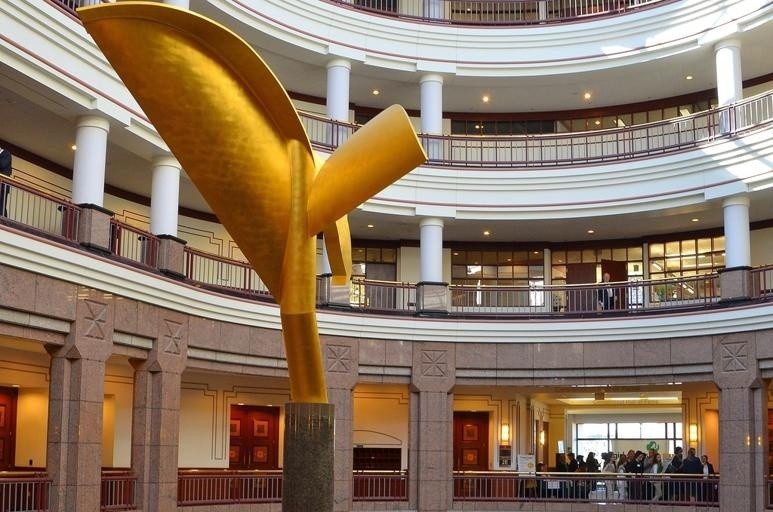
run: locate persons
[536,447,715,504]
[598,272,617,314]
